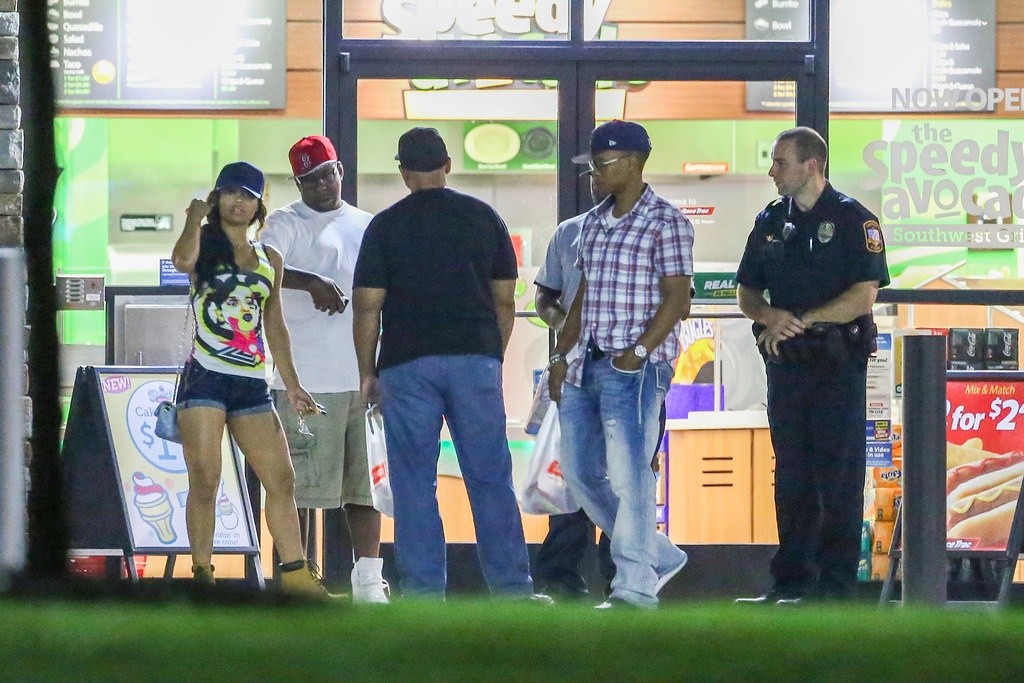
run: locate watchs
[793,307,805,321]
[632,343,650,361]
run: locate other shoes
[531,573,589,600]
[654,552,687,597]
[529,590,553,606]
[734,586,776,603]
[597,597,627,611]
[777,588,817,607]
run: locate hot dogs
[945,449,1024,548]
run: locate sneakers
[351,557,390,604]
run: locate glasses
[588,155,630,170]
[301,169,338,190]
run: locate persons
[172,162,348,600]
[351,127,557,603]
[259,136,389,602]
[533,154,666,601]
[548,121,696,610]
[736,128,890,608]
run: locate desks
[377,408,776,544]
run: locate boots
[282,565,350,604]
[193,564,217,584]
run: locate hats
[215,162,265,199]
[395,127,448,172]
[572,119,651,165]
[288,136,338,177]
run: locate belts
[593,348,604,359]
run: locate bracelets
[549,353,566,365]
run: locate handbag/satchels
[154,401,182,444]
[521,399,583,514]
[366,403,394,518]
